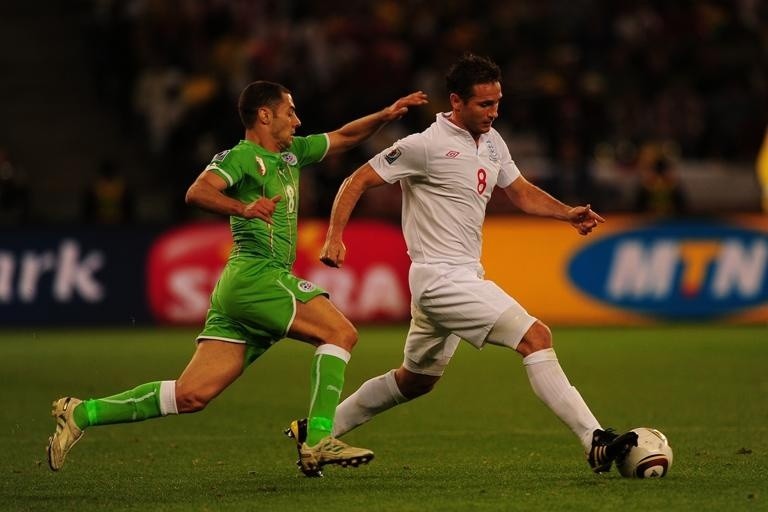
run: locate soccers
[616,427,673,479]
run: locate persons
[284,53,638,476]
[46,80,428,473]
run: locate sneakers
[299,435,374,477]
[585,428,638,472]
[46,396,85,471]
[283,417,323,477]
